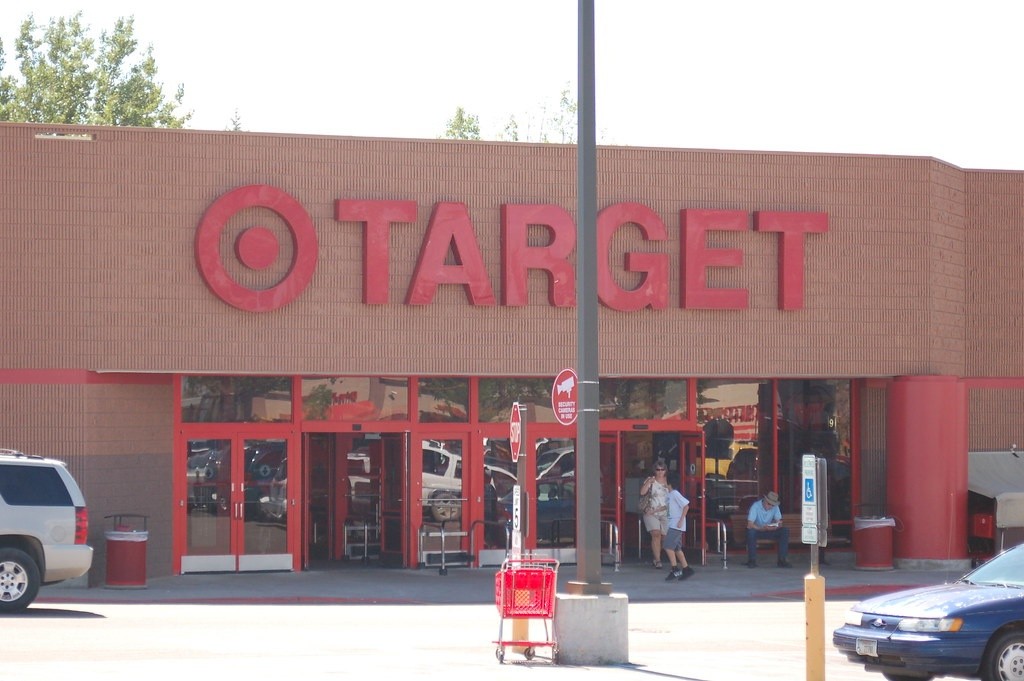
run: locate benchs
[731,513,831,567]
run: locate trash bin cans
[853,503,895,572]
[104,512,150,590]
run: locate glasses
[768,503,774,506]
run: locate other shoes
[778,561,792,568]
[653,560,662,568]
[747,560,756,568]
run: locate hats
[763,490,781,506]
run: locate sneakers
[665,568,681,580]
[678,566,695,581]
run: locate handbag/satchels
[639,477,653,513]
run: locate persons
[746,489,793,569]
[639,460,673,568]
[647,471,694,580]
